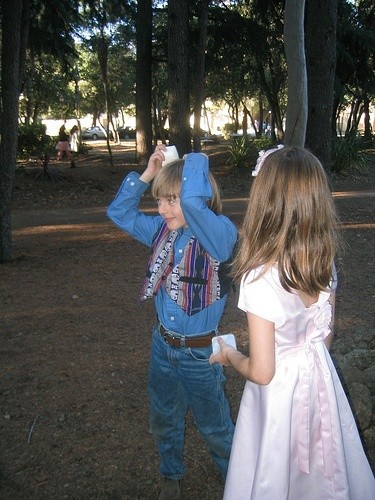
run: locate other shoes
[158,477,182,500]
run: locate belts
[157,320,217,348]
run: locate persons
[208,146,375,500]
[55,125,70,161]
[68,125,82,169]
[106,144,238,500]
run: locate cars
[82,125,107,140]
